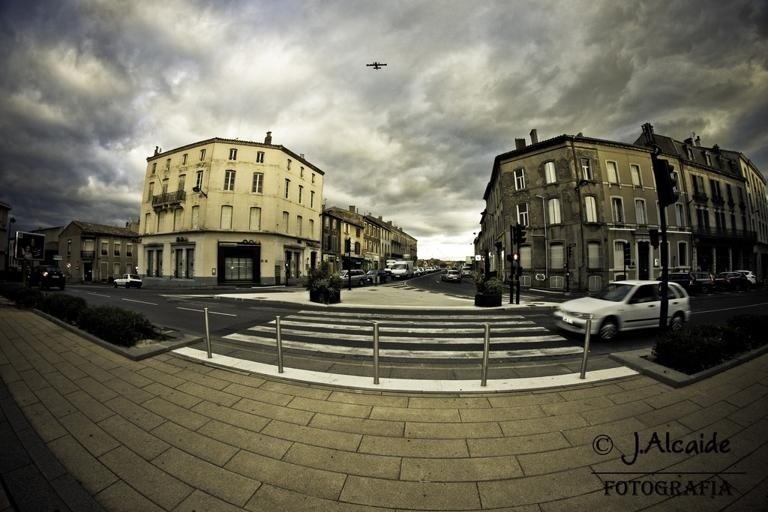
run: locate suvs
[26,263,68,293]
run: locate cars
[550,276,693,342]
[113,274,146,288]
[652,268,758,300]
[333,268,368,288]
[364,257,472,284]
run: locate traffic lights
[512,226,520,245]
[495,242,502,249]
[622,241,632,265]
[652,156,681,206]
[507,254,512,262]
[513,253,520,261]
[649,229,660,250]
[516,222,527,243]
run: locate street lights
[6,216,17,274]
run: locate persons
[21,243,34,258]
